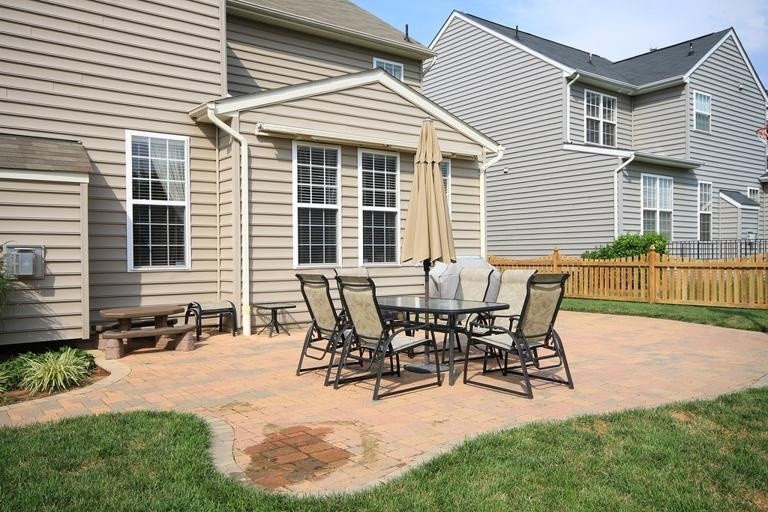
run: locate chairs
[295,267,574,399]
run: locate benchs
[103,324,197,360]
[95,317,178,350]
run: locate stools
[186,299,238,341]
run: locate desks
[99,304,184,350]
[254,302,297,337]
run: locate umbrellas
[401,115,457,374]
